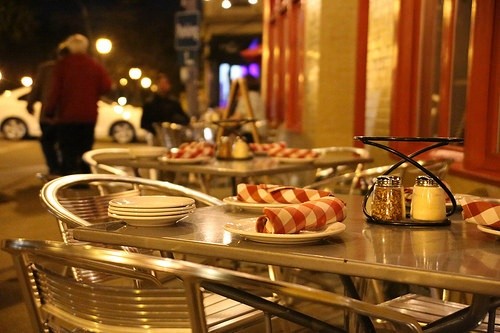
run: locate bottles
[370,175,405,222]
[218,135,247,158]
[410,175,447,221]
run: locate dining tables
[71,160,500,333]
[158,156,318,196]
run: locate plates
[446,193,480,209]
[255,151,267,155]
[156,155,210,163]
[273,157,316,163]
[222,217,346,244]
[215,155,254,161]
[108,196,196,227]
[477,224,500,236]
[222,196,298,214]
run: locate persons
[26,37,76,186]
[44,32,112,191]
[142,74,192,158]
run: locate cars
[0,87,143,145]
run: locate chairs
[0,146,500,333]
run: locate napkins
[171,141,215,158]
[250,142,287,153]
[268,148,319,158]
[237,183,333,205]
[257,194,348,236]
[462,201,500,229]
